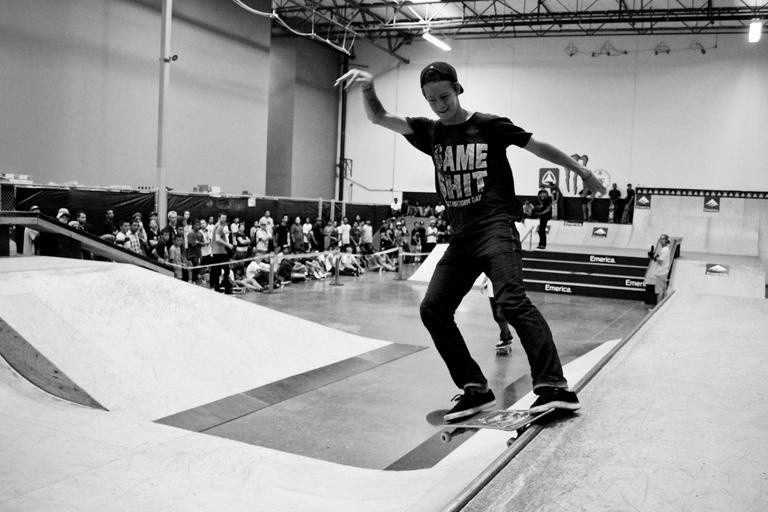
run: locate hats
[421,62,464,94]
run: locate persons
[330,60,607,423]
[8,197,455,296]
[479,272,514,356]
[646,234,671,312]
[517,184,635,249]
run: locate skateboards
[426,409,554,447]
[494,342,513,354]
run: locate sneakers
[444,388,496,421]
[529,389,582,415]
[496,340,511,347]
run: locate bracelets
[579,168,594,182]
